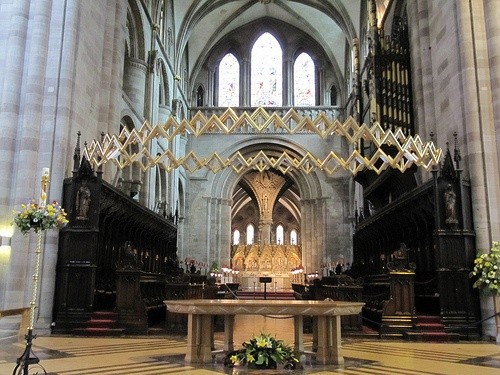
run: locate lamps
[184,258,239,277]
[290,261,344,278]
[0,235,12,246]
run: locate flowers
[8,199,69,237]
[467,240,500,297]
[223,328,305,370]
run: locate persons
[311,278,322,324]
[203,277,218,327]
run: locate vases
[247,358,278,370]
